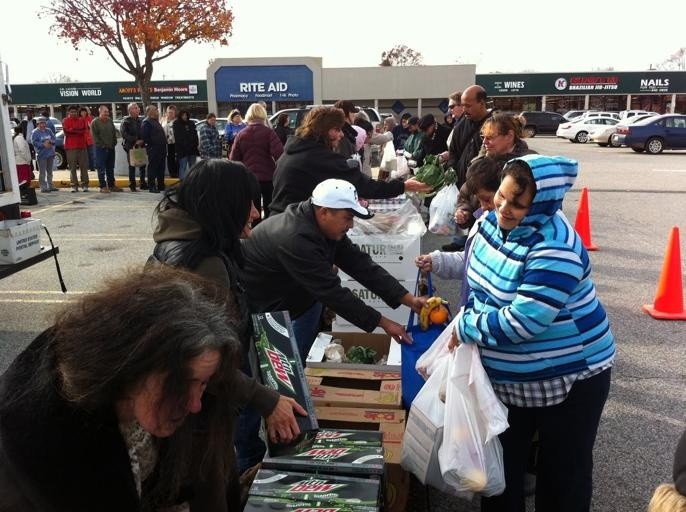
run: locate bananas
[419,297,449,332]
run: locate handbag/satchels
[399,321,457,408]
[19,187,38,206]
[128,143,150,168]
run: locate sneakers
[39,184,165,193]
[442,243,466,252]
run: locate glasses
[447,103,461,110]
[479,133,493,141]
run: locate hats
[418,113,435,130]
[308,177,370,218]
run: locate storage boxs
[330,197,428,331]
[0,217,42,264]
[243,310,406,511]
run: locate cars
[518,108,685,156]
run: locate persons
[647,430,686,512]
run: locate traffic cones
[642,227,686,323]
[573,188,601,253]
